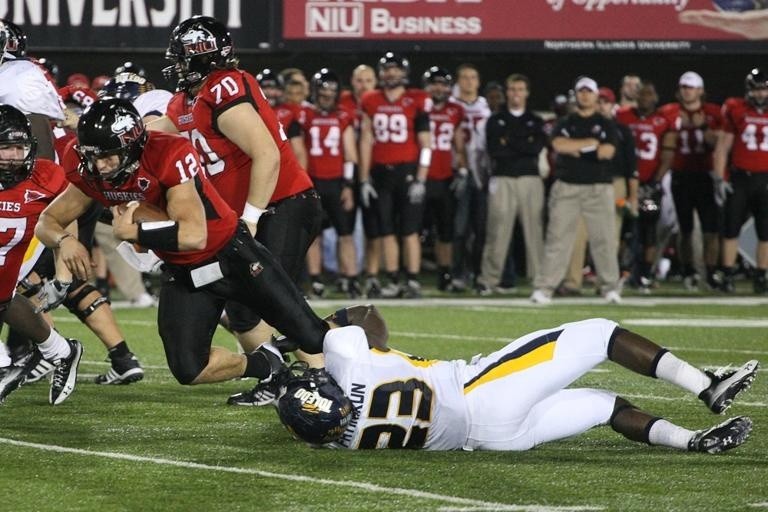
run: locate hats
[575,71,705,103]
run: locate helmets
[162,16,233,93]
[744,68,768,112]
[272,361,352,445]
[257,52,454,104]
[0,104,37,186]
[1,18,28,62]
[99,62,146,99]
[73,99,148,190]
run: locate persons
[268,304,758,470]
[255,49,767,305]
[0,13,345,407]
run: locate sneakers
[530,267,766,305]
[95,352,144,385]
[688,415,753,455]
[308,274,517,299]
[0,339,84,405]
[227,343,289,407]
[698,360,760,415]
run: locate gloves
[340,165,469,212]
[33,280,70,314]
[713,178,734,207]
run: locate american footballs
[118,201,168,222]
